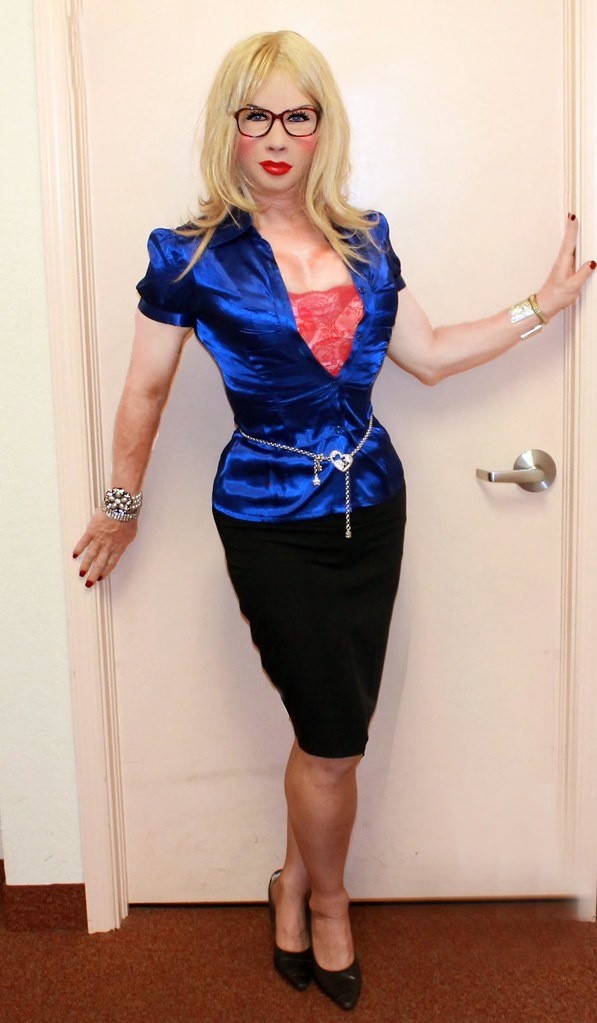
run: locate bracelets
[529,295,549,324]
[508,301,542,340]
[102,488,143,521]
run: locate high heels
[268,869,312,993]
[312,931,361,1011]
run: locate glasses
[233,107,322,138]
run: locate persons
[71,32,596,1010]
